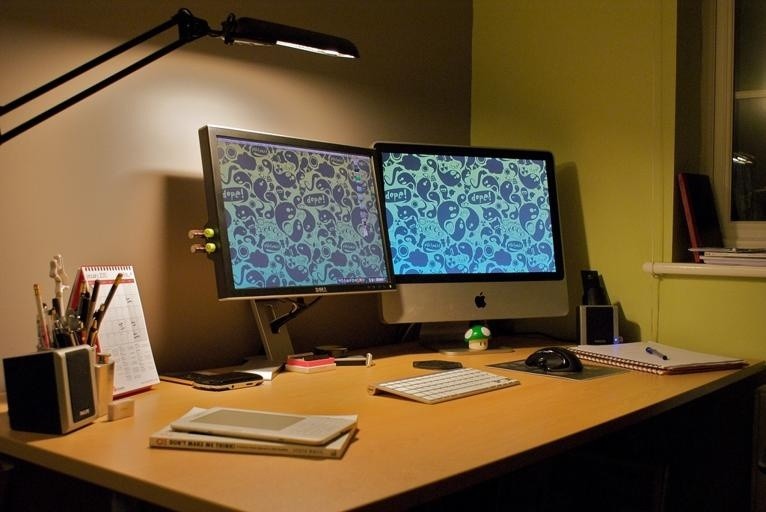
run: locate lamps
[0,7,360,144]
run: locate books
[678,169,766,267]
[574,336,752,379]
[147,406,360,463]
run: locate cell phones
[413,359,463,371]
[194,372,262,391]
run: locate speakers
[2,343,99,435]
[575,304,619,346]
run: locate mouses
[524,346,584,372]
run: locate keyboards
[367,367,521,405]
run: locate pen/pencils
[646,346,668,360]
[33,254,123,363]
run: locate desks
[2,332,762,510]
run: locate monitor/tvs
[197,124,397,381]
[370,140,570,356]
